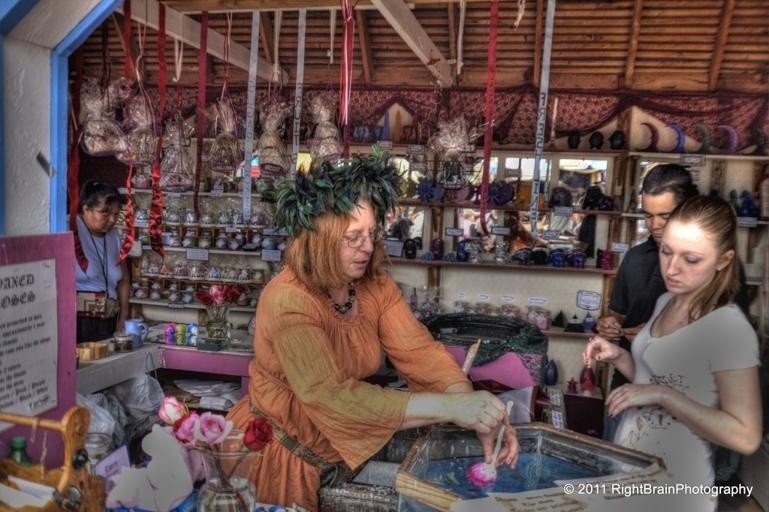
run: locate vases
[185,432,263,510]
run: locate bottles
[137,207,288,281]
[7,437,32,466]
[114,334,134,353]
[79,432,114,480]
[454,294,552,331]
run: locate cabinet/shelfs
[102,142,768,400]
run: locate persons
[582,195,764,512]
[214,144,520,512]
[579,185,601,246]
[592,165,750,445]
[65,179,132,346]
[536,187,575,234]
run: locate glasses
[342,228,385,248]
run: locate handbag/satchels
[76,291,108,319]
[316,481,400,512]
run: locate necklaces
[320,278,356,313]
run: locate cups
[124,319,149,348]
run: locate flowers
[154,392,273,510]
